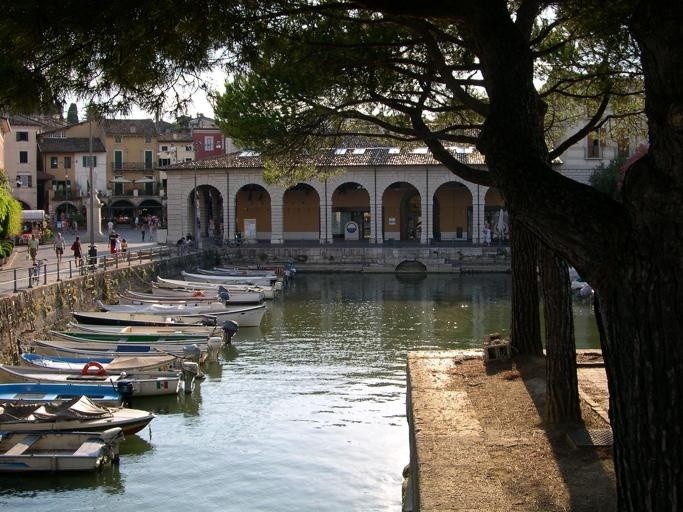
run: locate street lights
[64,172,68,212]
[160,192,168,230]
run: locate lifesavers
[192,291,206,297]
[83,362,107,376]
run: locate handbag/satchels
[26,250,30,261]
[70,241,76,250]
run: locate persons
[183,236,192,244]
[72,236,82,268]
[106,231,116,253]
[133,214,160,241]
[59,212,79,234]
[27,235,39,263]
[53,233,65,262]
[102,214,129,233]
[119,238,128,252]
[176,237,185,245]
[113,233,120,252]
